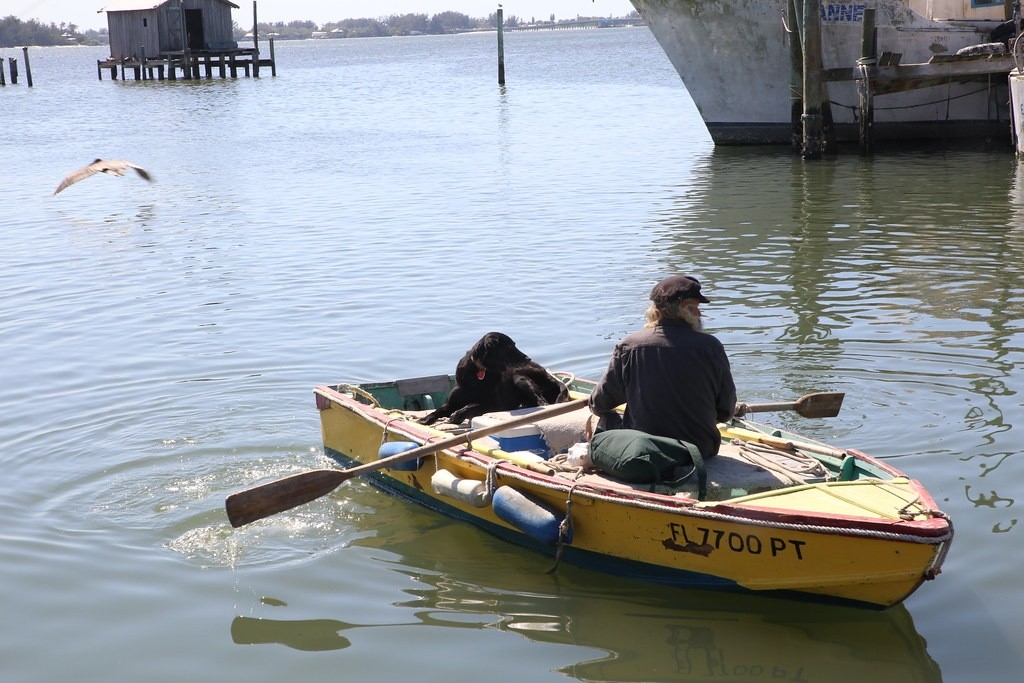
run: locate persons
[589,276,737,459]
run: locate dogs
[417,332,561,426]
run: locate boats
[312,364,952,615]
[628,0,1024,149]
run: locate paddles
[733,392,846,419]
[223,398,590,530]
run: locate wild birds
[53,157,154,195]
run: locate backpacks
[589,430,707,501]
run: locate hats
[649,275,711,303]
